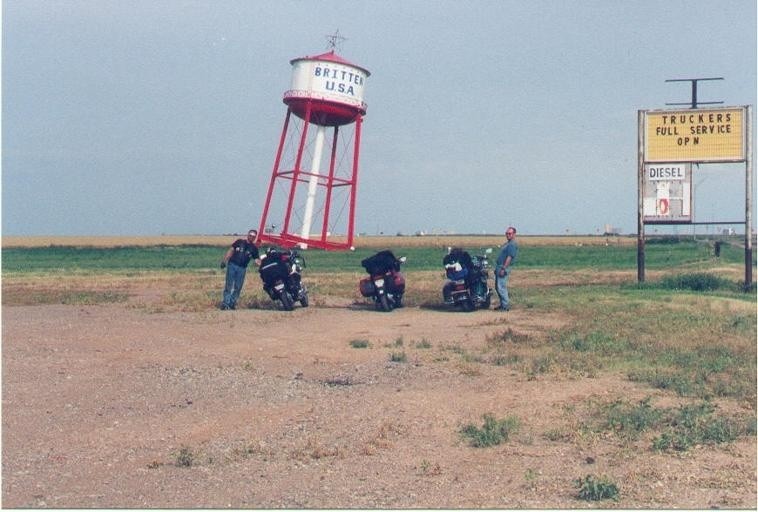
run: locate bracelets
[502,267,505,270]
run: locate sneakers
[221,303,235,310]
[494,305,509,312]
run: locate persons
[220,230,261,310]
[494,227,518,311]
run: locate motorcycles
[258,244,311,310]
[442,246,494,311]
[359,250,407,313]
